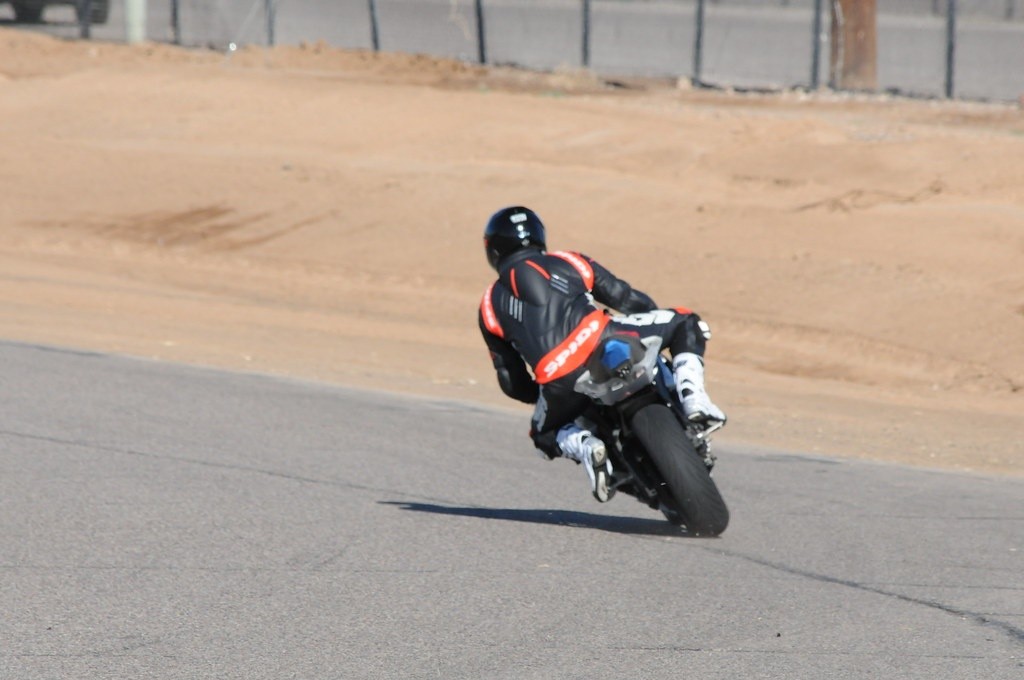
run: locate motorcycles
[573,333,729,538]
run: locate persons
[478,206,728,503]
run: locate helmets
[484,206,546,268]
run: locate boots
[673,352,727,432]
[556,423,616,503]
